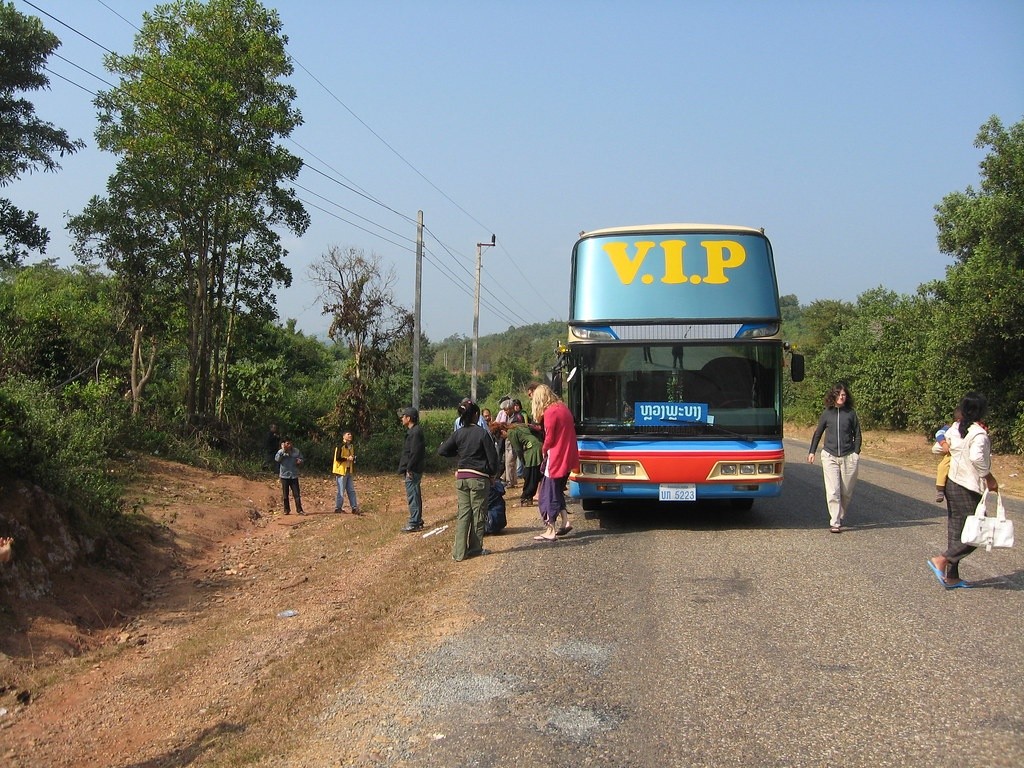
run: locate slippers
[534,534,556,541]
[556,526,575,538]
[947,580,975,589]
[928,560,948,588]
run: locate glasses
[528,392,533,396]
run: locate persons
[397,407,424,532]
[935,406,963,502]
[926,391,998,587]
[277,437,307,517]
[450,381,538,498]
[333,431,362,514]
[530,383,578,541]
[437,400,498,562]
[807,382,862,533]
[488,420,542,508]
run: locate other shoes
[283,511,290,515]
[351,507,365,515]
[937,490,944,503]
[334,510,347,515]
[298,511,308,516]
[401,525,422,532]
[512,498,534,507]
[830,526,841,533]
[505,483,517,489]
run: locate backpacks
[484,479,507,535]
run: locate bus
[550,224,806,512]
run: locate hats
[402,407,419,420]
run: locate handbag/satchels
[526,422,545,440]
[960,486,1014,552]
